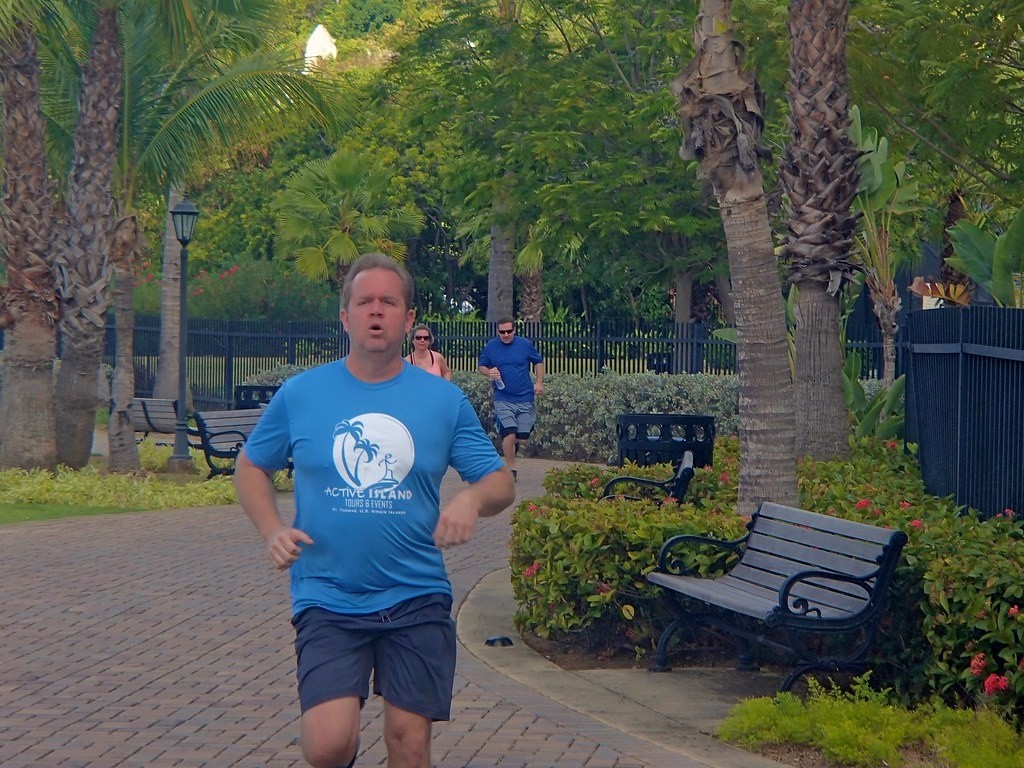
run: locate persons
[478,316,544,482]
[234,251,517,768]
[404,324,451,381]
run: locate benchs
[646,501,907,693]
[133,398,202,449]
[193,408,294,481]
[600,451,695,502]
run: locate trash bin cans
[616,412,715,468]
[234,384,282,410]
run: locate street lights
[156,192,199,476]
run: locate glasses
[497,328,514,335]
[414,335,429,341]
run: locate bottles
[495,378,504,390]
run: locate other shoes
[515,443,520,455]
[511,469,518,483]
